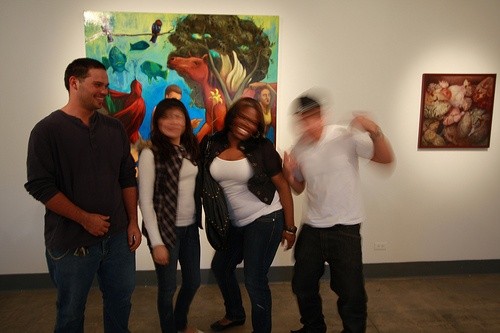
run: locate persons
[24,58,142,333]
[136,98,204,333]
[137,97,297,332]
[283,97,393,333]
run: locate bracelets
[286,231,293,234]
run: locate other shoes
[291,325,327,333]
[178,327,205,333]
[210,317,245,331]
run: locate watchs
[286,225,297,233]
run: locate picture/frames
[417,74,497,148]
